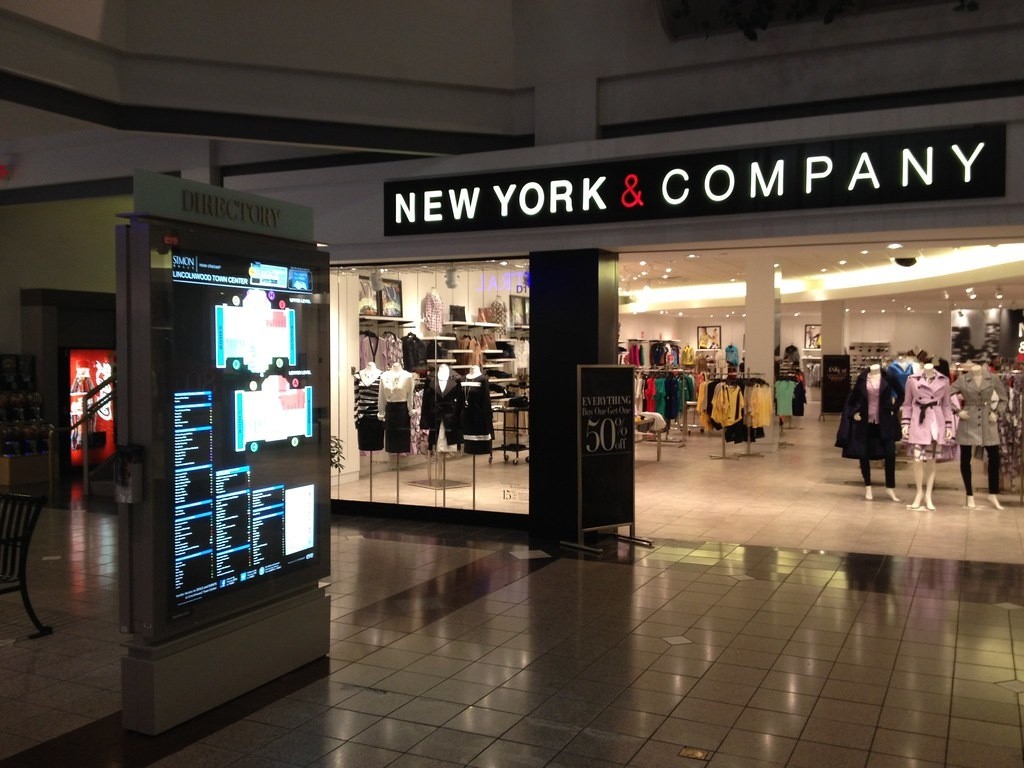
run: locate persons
[352,360,495,455]
[489,295,507,336]
[847,357,1007,513]
[422,286,444,332]
[806,326,821,348]
[698,327,719,349]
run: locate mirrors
[949,307,1002,365]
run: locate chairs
[0,494,53,634]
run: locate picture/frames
[805,324,822,349]
[358,276,380,316]
[697,326,721,349]
[380,279,403,317]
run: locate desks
[634,415,663,462]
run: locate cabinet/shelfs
[488,406,530,464]
[676,399,706,436]
[421,321,516,398]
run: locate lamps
[444,262,458,289]
[994,288,1005,300]
[965,287,976,300]
[370,268,385,291]
